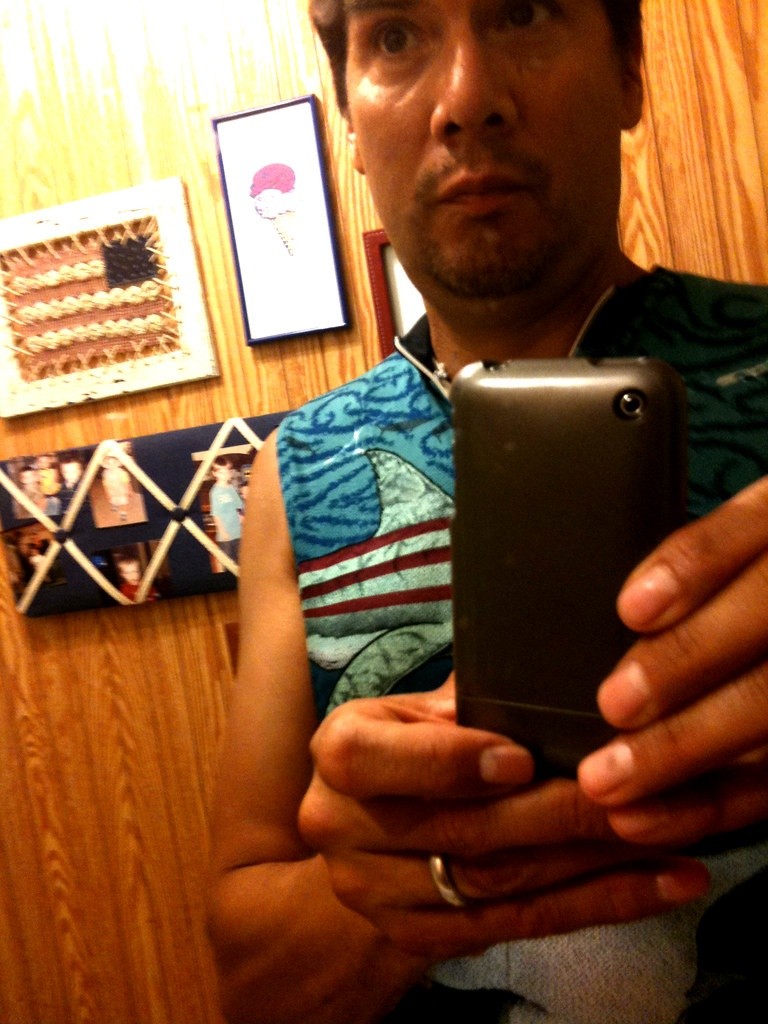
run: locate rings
[428,853,465,913]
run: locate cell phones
[450,358,686,789]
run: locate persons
[12,455,252,603]
[202,0,768,1024]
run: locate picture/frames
[213,94,351,346]
[0,176,221,427]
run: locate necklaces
[431,354,452,384]
